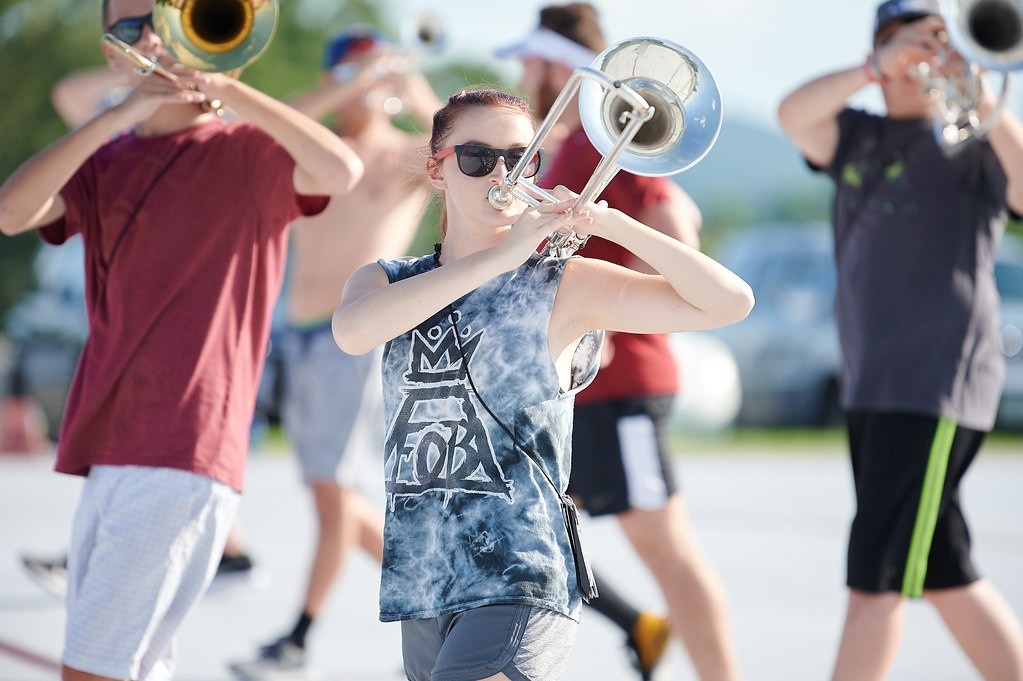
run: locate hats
[321,26,385,72]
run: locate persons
[0,62,110,575]
[215,27,443,680]
[491,4,740,680]
[779,0,1023,680]
[0,0,363,681]
[334,90,756,680]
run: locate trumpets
[906,1,1023,156]
[486,32,726,263]
[97,0,284,121]
[358,14,453,115]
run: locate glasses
[432,144,541,178]
[106,12,154,46]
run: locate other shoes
[218,555,255,585]
[22,557,70,597]
[625,612,673,681]
[227,638,310,681]
